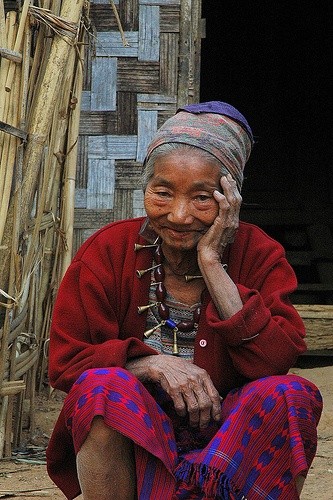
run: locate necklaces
[129,219,230,355]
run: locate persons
[45,105,324,500]
